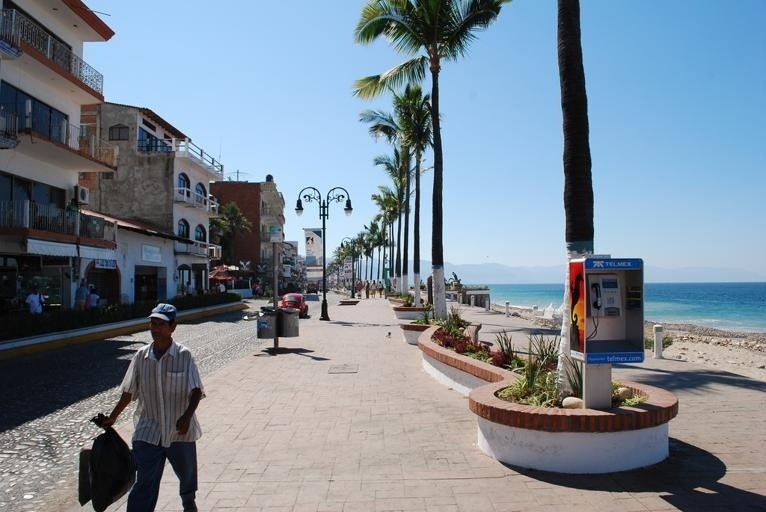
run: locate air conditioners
[72,183,91,206]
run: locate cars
[279,292,308,318]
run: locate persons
[356,280,383,298]
[95,303,204,512]
[183,281,192,296]
[75,284,100,313]
[24,285,45,335]
[217,281,225,293]
[252,282,262,298]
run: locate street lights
[294,186,352,320]
[341,235,356,298]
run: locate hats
[148,303,176,323]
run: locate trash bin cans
[278,308,300,337]
[241,306,276,338]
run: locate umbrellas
[208,268,234,285]
